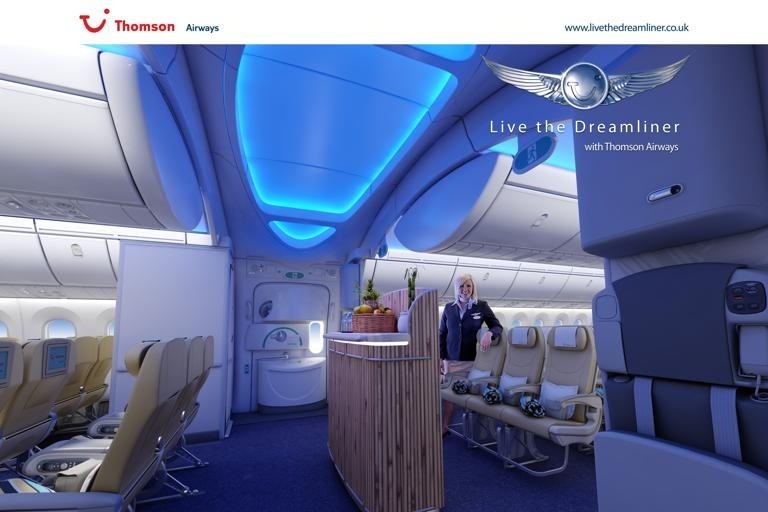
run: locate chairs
[436,323,603,478]
[0,335,214,510]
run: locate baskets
[350,312,397,333]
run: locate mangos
[353,304,393,315]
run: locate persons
[438,271,504,441]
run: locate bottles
[341,312,352,333]
